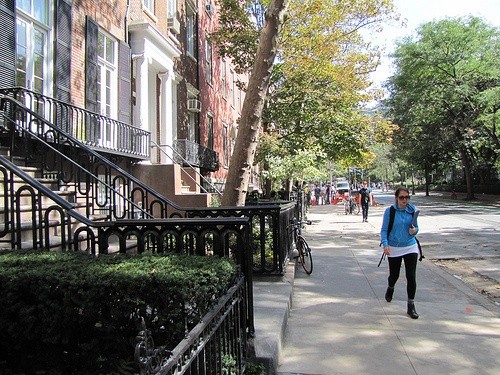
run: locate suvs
[334,182,349,195]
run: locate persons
[380,185,419,319]
[359,181,374,222]
[301,181,387,215]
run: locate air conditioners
[206,4,215,15]
[187,99,202,113]
[167,16,180,35]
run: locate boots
[385,285,394,302]
[407,301,418,319]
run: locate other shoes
[365,220,368,222]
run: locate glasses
[398,196,410,200]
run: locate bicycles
[345,197,360,215]
[285,217,313,276]
